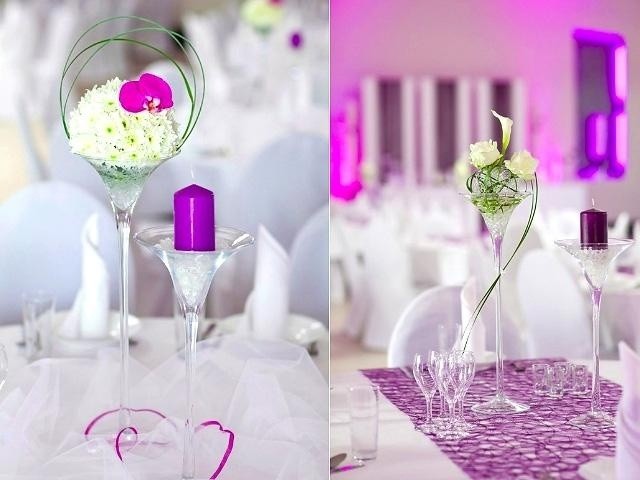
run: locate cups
[22,293,54,360]
[348,386,379,461]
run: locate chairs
[0,59,329,341]
[329,184,640,480]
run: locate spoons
[330,451,347,469]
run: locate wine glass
[70,145,256,478]
[414,192,635,440]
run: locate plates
[52,309,143,352]
[220,313,327,348]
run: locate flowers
[457,110,538,354]
[58,12,206,184]
